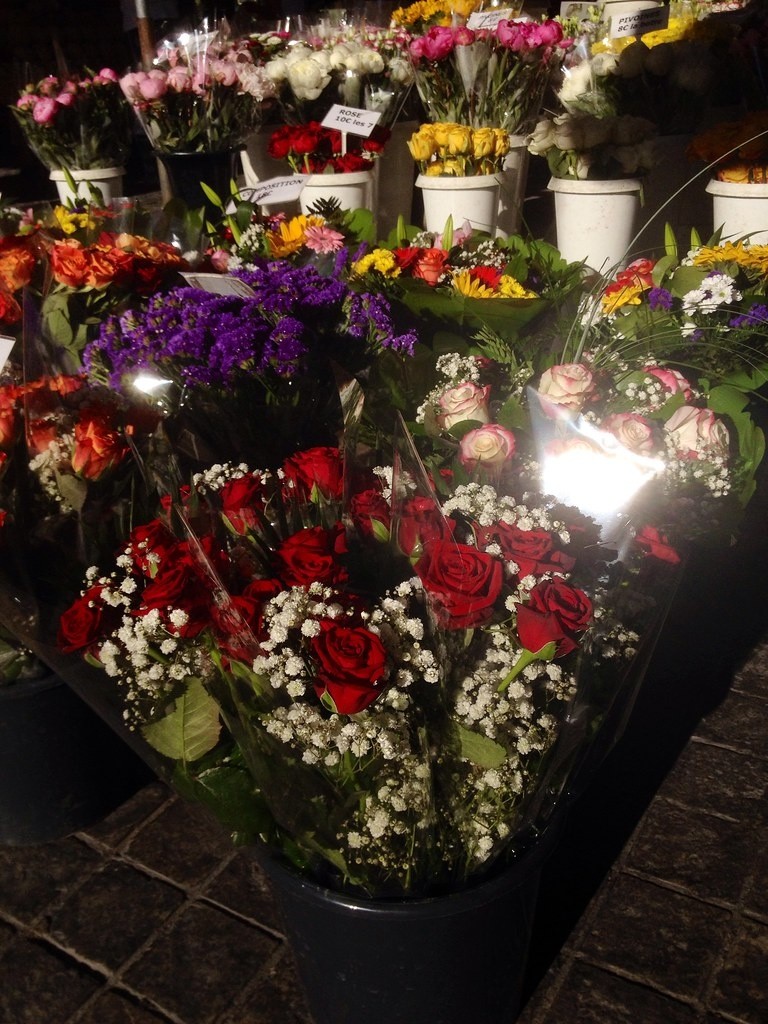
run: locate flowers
[0,1,768,899]
